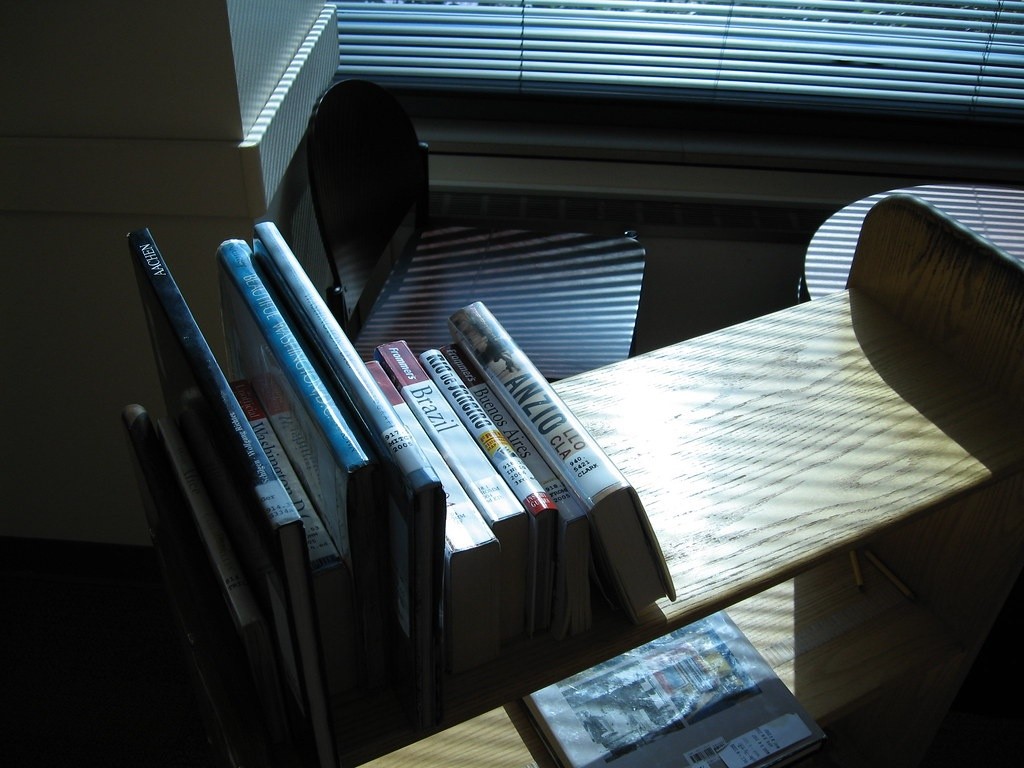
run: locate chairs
[306,80,647,382]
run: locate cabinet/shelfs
[121,191,1024,768]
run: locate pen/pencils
[864,549,916,601]
[849,551,865,592]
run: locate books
[522,610,825,768]
[129,220,675,768]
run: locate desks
[798,184,1024,303]
